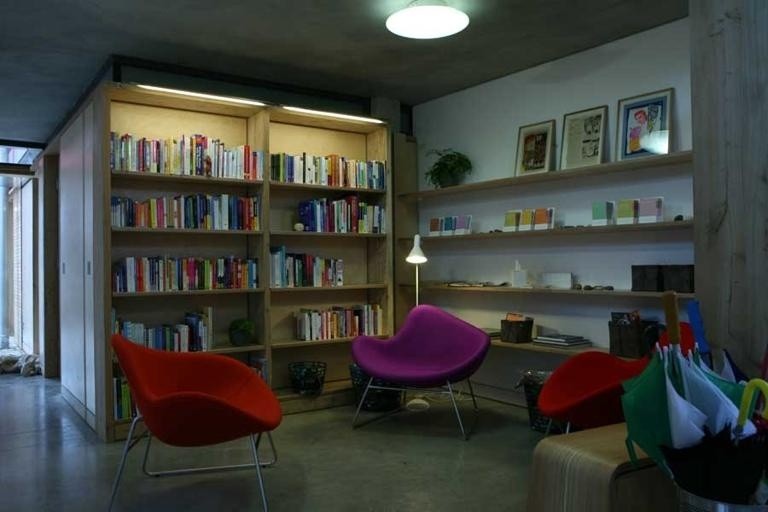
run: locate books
[110,130,264,179]
[111,256,269,421]
[269,245,343,287]
[297,303,386,341]
[298,197,386,233]
[532,334,592,347]
[479,328,502,340]
[503,207,556,232]
[592,195,666,228]
[428,215,473,238]
[271,151,385,190]
[111,193,261,230]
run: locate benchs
[526,421,680,512]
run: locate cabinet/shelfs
[397,150,693,362]
[93,79,267,443]
[262,104,393,416]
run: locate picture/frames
[614,85,674,162]
[513,119,554,176]
[558,104,607,170]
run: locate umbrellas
[617,284,768,512]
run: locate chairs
[350,304,490,441]
[535,322,694,439]
[106,333,282,512]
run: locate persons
[629,109,652,155]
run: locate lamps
[384,0,470,39]
[403,234,429,306]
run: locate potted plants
[227,317,258,346]
[423,146,472,191]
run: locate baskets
[289,362,326,397]
[500,317,533,343]
[631,264,661,292]
[608,320,657,358]
[660,265,694,293]
[349,363,401,411]
[522,369,573,433]
